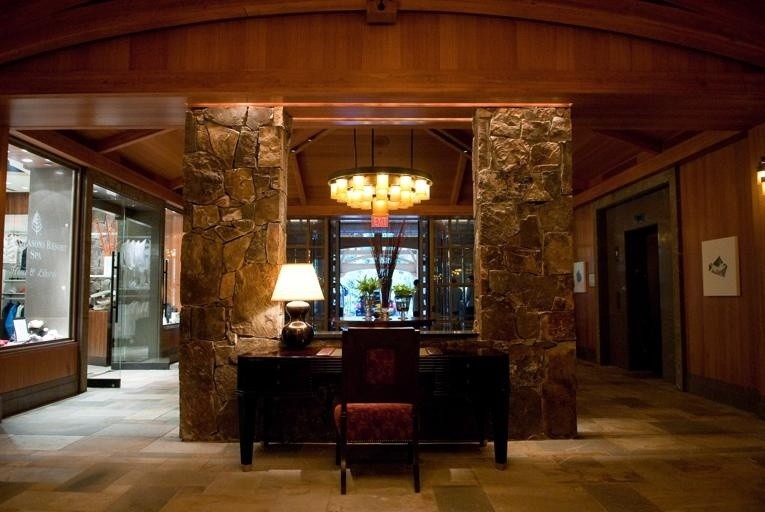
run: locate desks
[236,343,511,472]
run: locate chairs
[332,324,428,493]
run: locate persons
[412,275,474,317]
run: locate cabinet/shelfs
[285,217,474,331]
[2,278,27,295]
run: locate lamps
[270,261,324,347]
[325,126,434,216]
[756,155,765,198]
